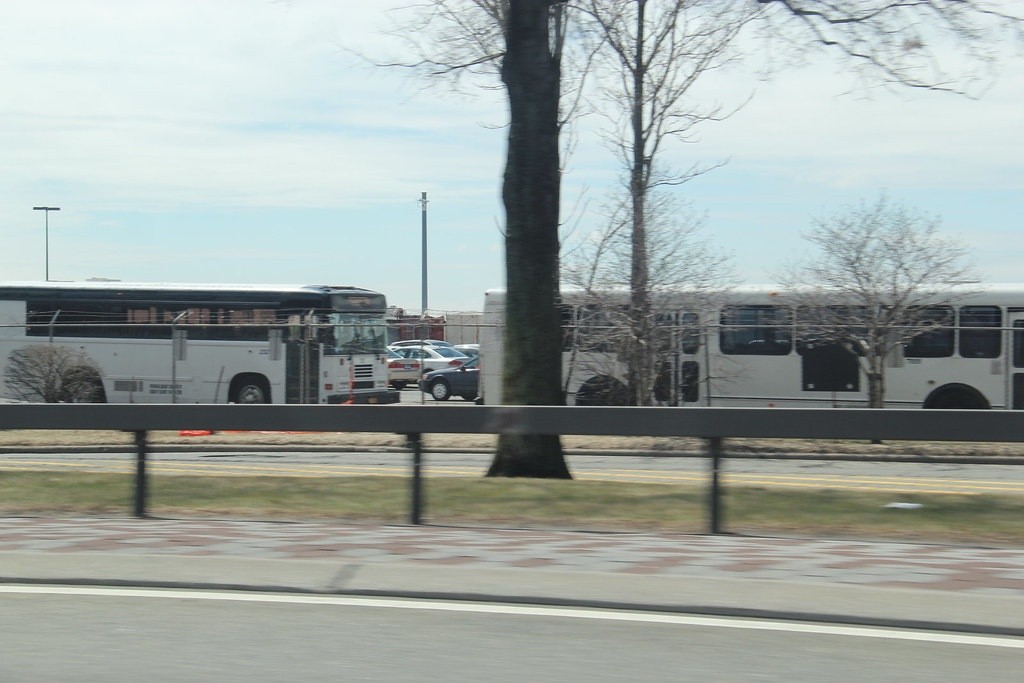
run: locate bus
[0,286,390,404]
[477,288,1024,410]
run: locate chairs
[411,351,418,358]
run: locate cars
[387,338,480,402]
